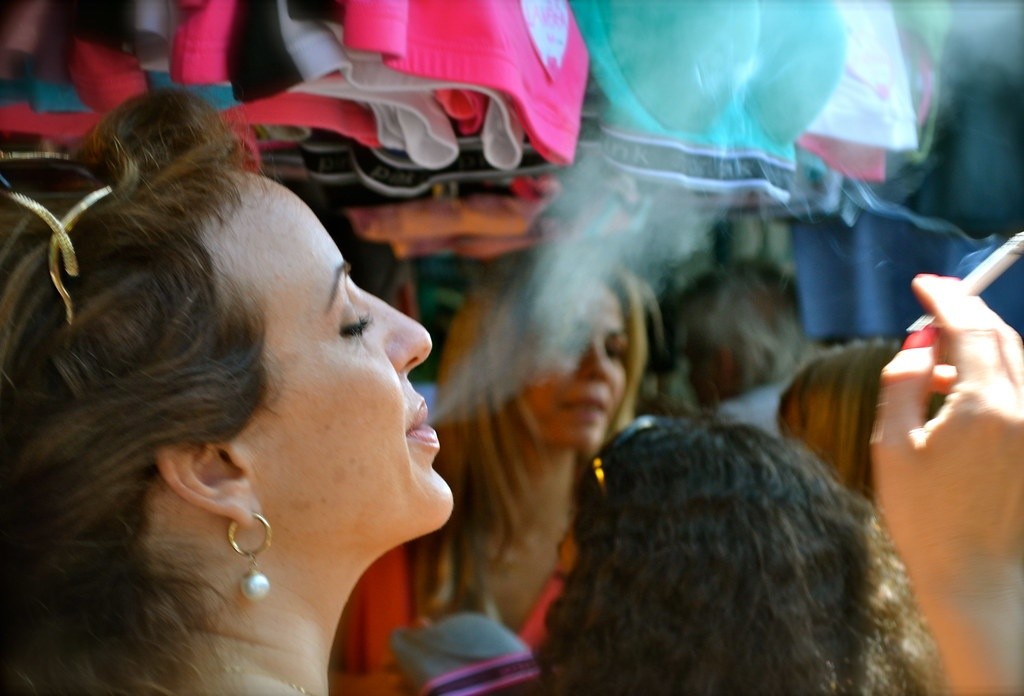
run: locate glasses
[591,414,692,494]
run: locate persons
[532,412,945,696]
[326,245,648,696]
[684,260,808,431]
[778,338,903,502]
[0,87,1024,696]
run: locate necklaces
[222,667,310,696]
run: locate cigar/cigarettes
[961,231,1024,297]
